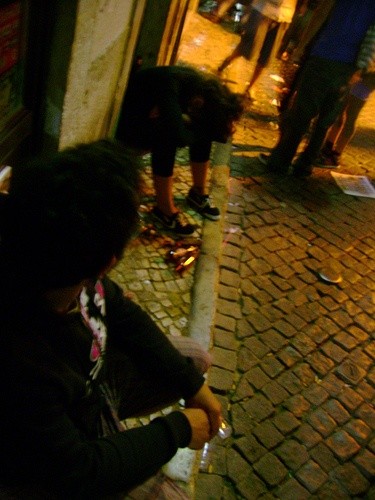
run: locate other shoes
[258,153,276,168]
[287,161,308,177]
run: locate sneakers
[151,205,195,236]
[185,187,221,221]
[312,148,341,168]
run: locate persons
[0,140,223,500]
[115,66,243,236]
[215,0,375,178]
